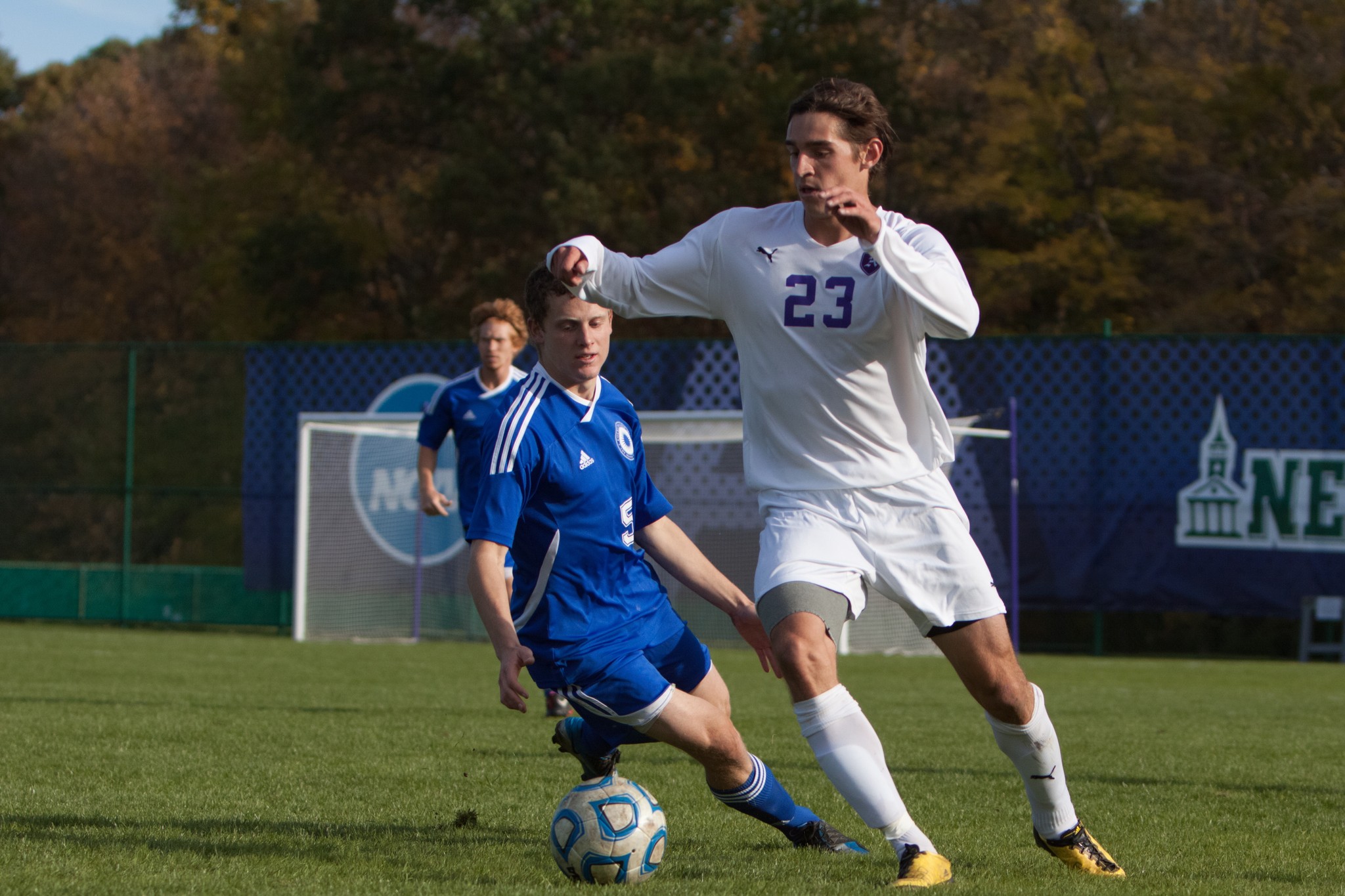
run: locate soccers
[550,776,667,883]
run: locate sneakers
[885,843,952,889]
[793,820,870,855]
[1033,818,1126,878]
[552,716,621,781]
[546,692,571,717]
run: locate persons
[463,258,874,862]
[413,298,574,720]
[543,79,1131,890]
